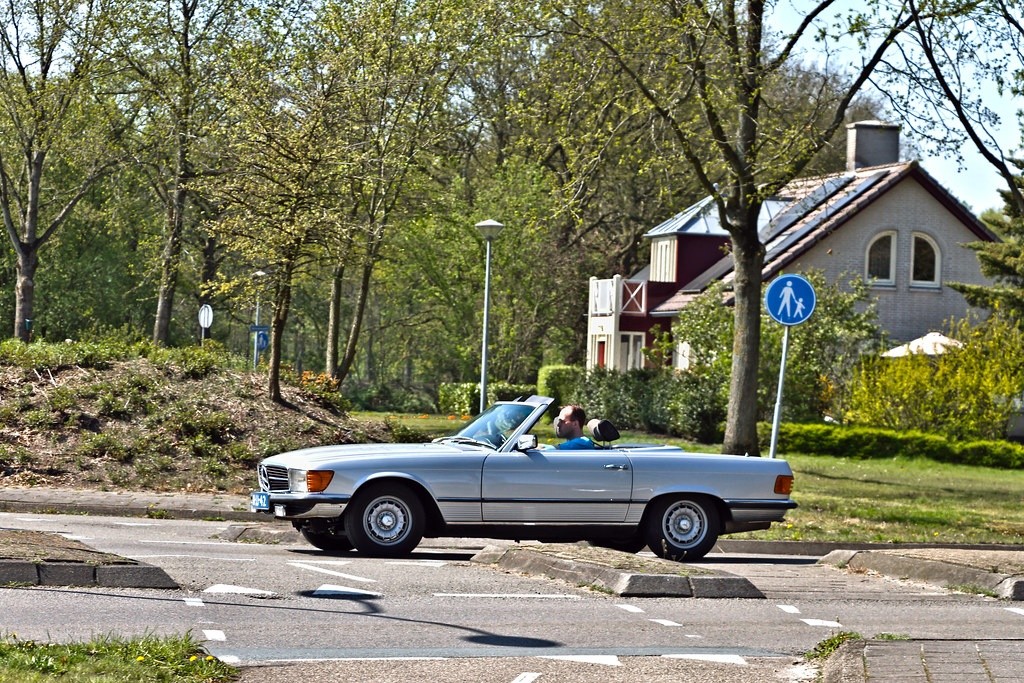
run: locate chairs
[587,417,620,447]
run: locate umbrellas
[882,332,977,358]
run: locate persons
[553,406,594,450]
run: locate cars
[252,394,798,558]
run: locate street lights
[474,219,506,412]
